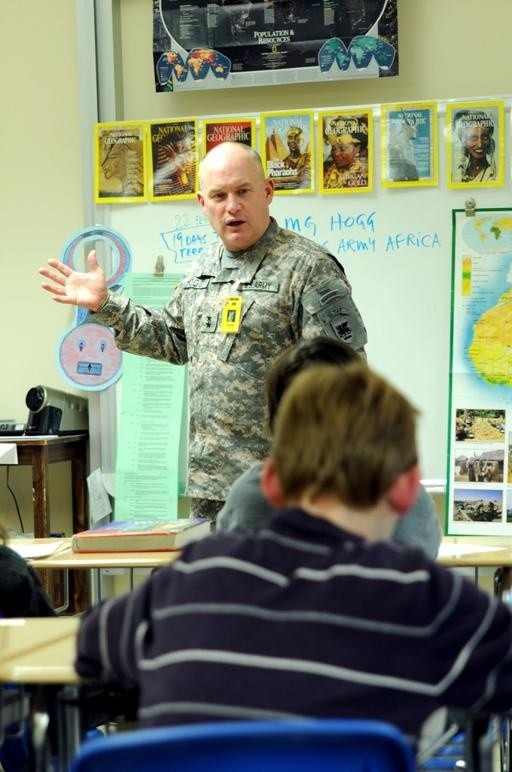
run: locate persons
[479,499,484,514]
[466,455,476,482]
[204,325,447,563]
[492,500,498,511]
[39,138,368,522]
[0,518,67,772]
[478,459,484,471]
[321,117,368,189]
[269,126,311,191]
[72,360,511,769]
[449,108,496,182]
[102,135,143,195]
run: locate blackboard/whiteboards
[91,97,512,493]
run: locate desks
[0,535,512,771]
[0,432,91,615]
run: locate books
[70,513,214,554]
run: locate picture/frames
[444,98,507,193]
[147,117,201,205]
[201,117,257,162]
[93,119,148,205]
[377,98,439,191]
[314,104,376,198]
[257,106,316,200]
